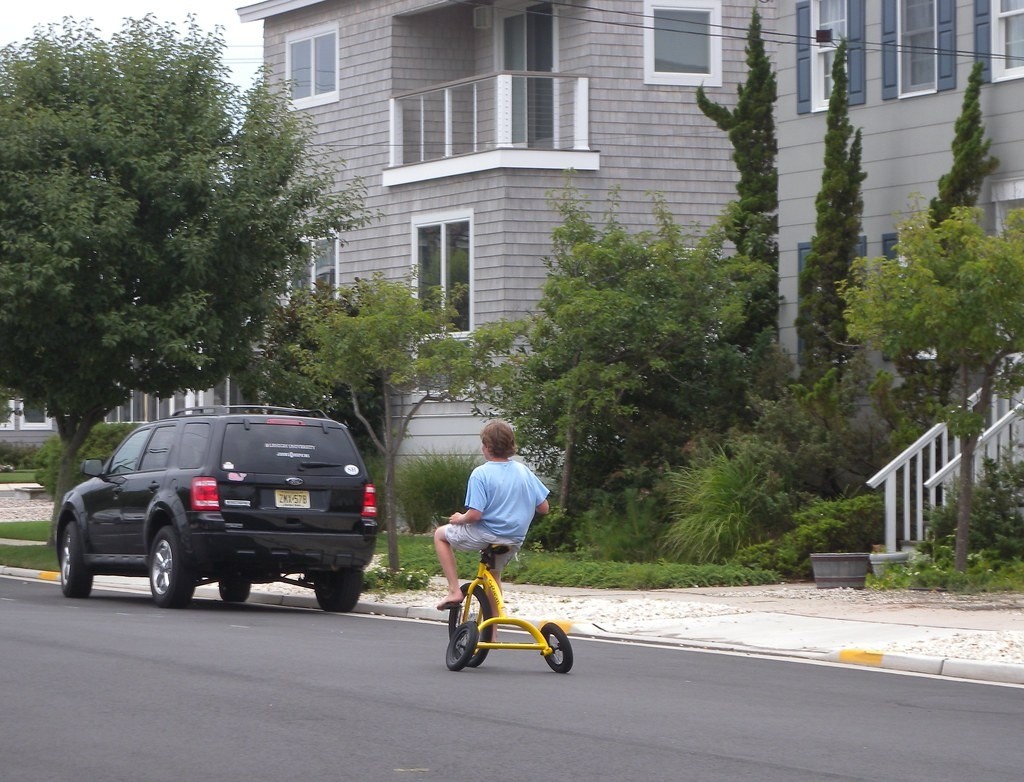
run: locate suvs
[56,404,378,607]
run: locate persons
[434,421,551,643]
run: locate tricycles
[443,514,573,674]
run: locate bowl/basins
[810,552,870,589]
